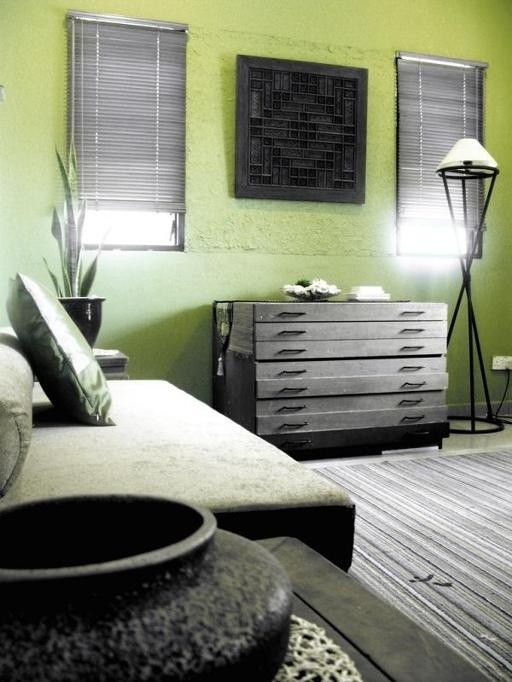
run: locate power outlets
[492,355,512,371]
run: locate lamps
[430,137,505,434]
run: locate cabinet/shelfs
[212,301,448,460]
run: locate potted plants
[42,137,107,351]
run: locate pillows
[5,272,117,427]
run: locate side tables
[84,348,128,379]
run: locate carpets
[302,448,512,682]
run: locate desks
[247,534,494,682]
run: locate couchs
[0,324,351,573]
